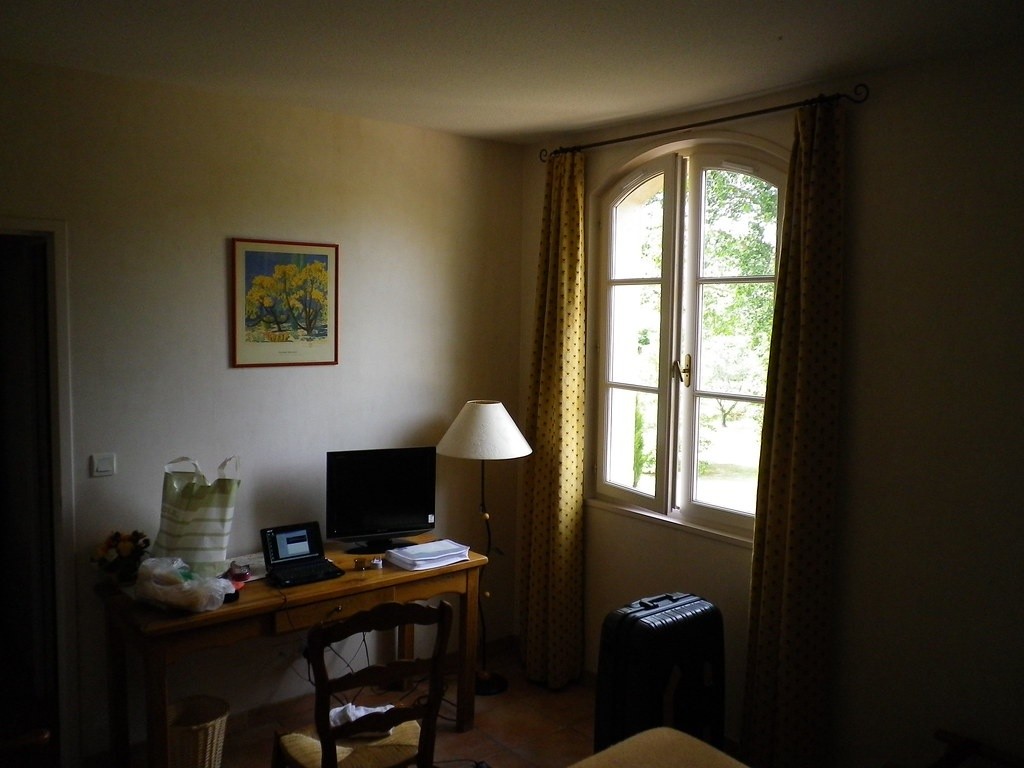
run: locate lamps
[436,400,534,697]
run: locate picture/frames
[230,237,339,369]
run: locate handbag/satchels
[151,455,241,579]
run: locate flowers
[89,529,150,575]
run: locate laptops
[260,520,345,589]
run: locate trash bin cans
[146,695,232,768]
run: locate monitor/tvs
[326,446,438,554]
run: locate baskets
[168,694,231,768]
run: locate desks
[92,531,489,768]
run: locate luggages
[594,592,725,754]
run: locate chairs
[270,600,454,768]
[566,726,751,768]
